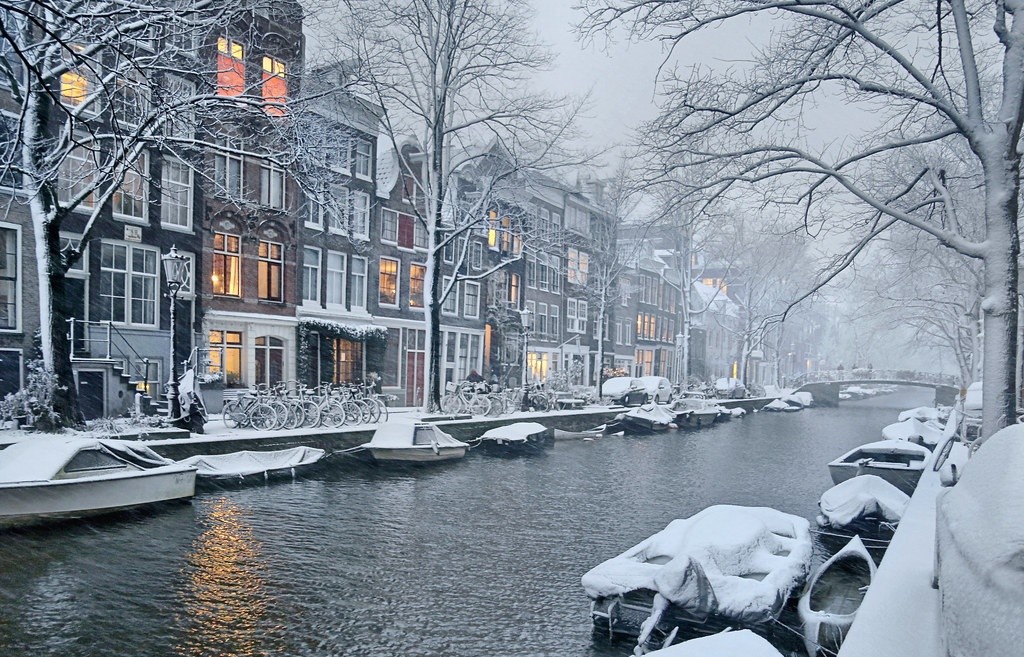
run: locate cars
[708,378,745,399]
[932,420,1024,656]
[747,383,766,397]
[602,377,648,406]
[641,376,672,404]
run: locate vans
[959,379,984,441]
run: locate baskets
[446,382,459,393]
[493,384,500,393]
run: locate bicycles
[222,380,389,431]
[440,381,552,416]
[931,407,983,471]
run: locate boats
[581,505,813,641]
[671,392,722,427]
[782,392,815,408]
[828,439,932,496]
[0,437,199,527]
[176,446,330,486]
[717,406,747,420]
[615,404,673,433]
[552,424,608,440]
[881,418,945,452]
[764,397,790,412]
[475,422,555,454]
[362,422,471,460]
[815,475,911,533]
[797,534,877,657]
[838,386,897,401]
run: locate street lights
[160,244,187,427]
[519,305,534,412]
[311,330,320,396]
[673,332,683,393]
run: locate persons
[852,364,857,369]
[867,363,873,369]
[837,364,844,379]
[467,370,484,382]
[489,370,498,384]
